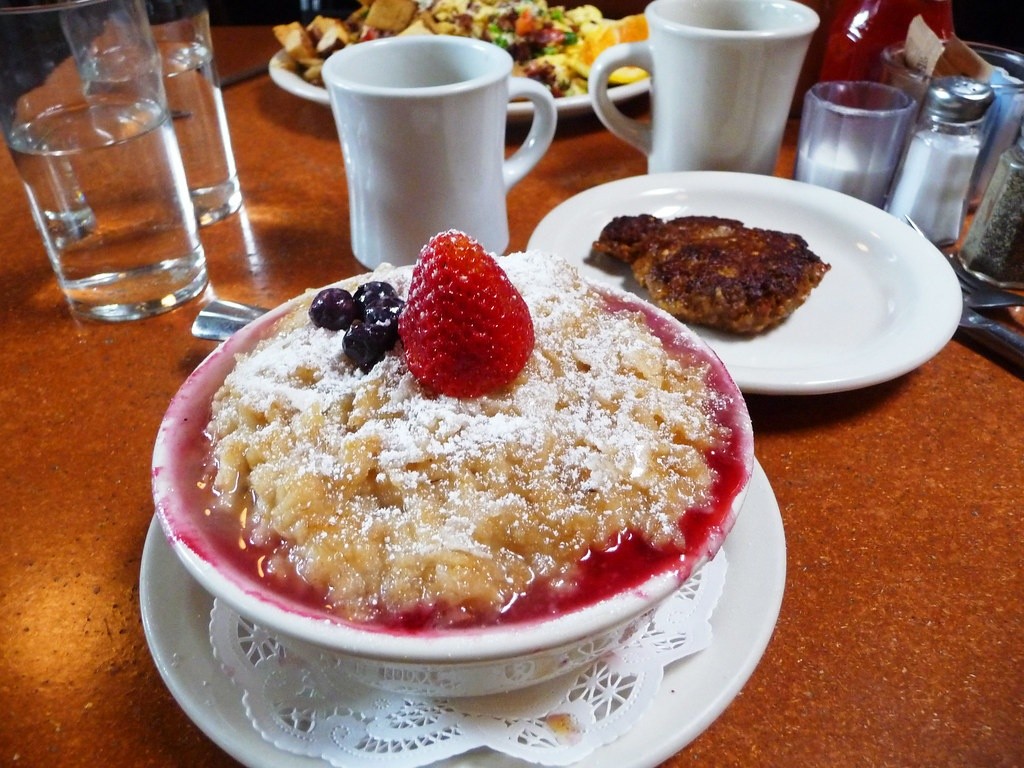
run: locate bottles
[959,125,1024,288]
[820,0,955,126]
[883,76,996,244]
[779,0,828,131]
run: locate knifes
[959,296,1024,369]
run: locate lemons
[564,14,655,85]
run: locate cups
[0,0,209,320]
[795,81,918,208]
[589,0,820,176]
[322,38,556,271]
[59,0,241,223]
[878,40,1024,206]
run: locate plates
[268,17,650,122]
[138,455,787,768]
[527,172,963,394]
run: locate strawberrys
[396,227,536,398]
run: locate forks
[939,248,1024,312]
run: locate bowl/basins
[152,265,753,699]
